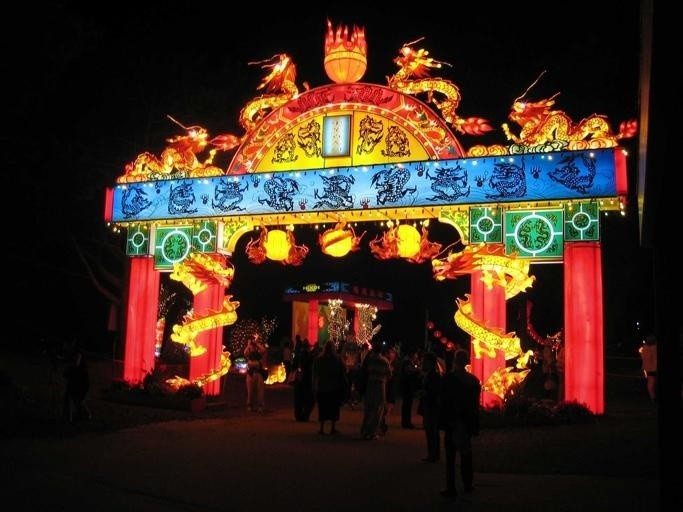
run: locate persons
[397,357,422,429]
[519,348,560,398]
[418,351,442,466]
[282,335,312,382]
[294,337,399,441]
[61,350,90,436]
[436,347,481,500]
[243,337,267,416]
[639,335,656,405]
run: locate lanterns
[440,337,448,344]
[433,331,442,337]
[427,324,434,330]
[446,342,454,348]
